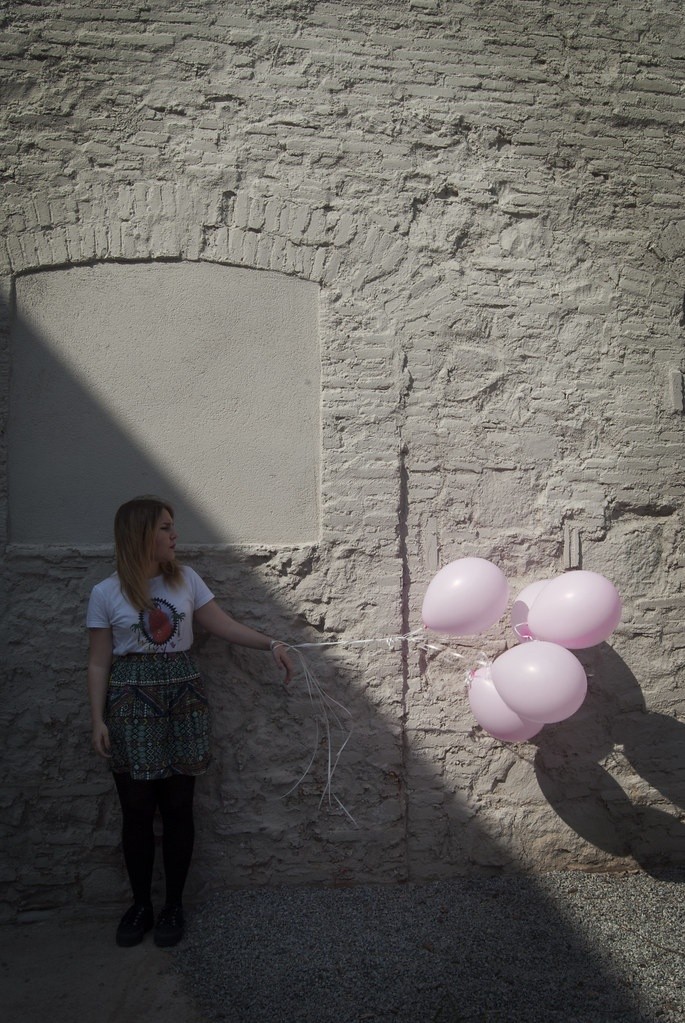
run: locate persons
[85,494,294,947]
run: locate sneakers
[116,902,154,947]
[154,903,184,946]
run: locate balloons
[421,557,509,637]
[490,640,587,723]
[510,580,550,641]
[529,569,622,649]
[466,668,544,744]
